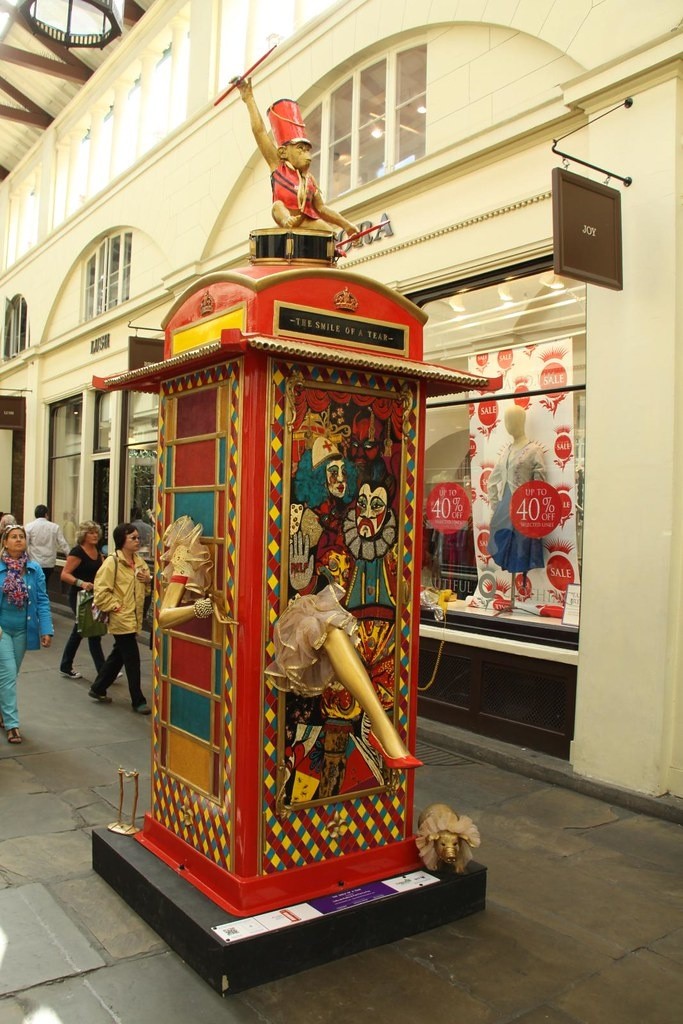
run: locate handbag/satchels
[91,554,118,623]
[75,590,107,637]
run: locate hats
[0,514,17,529]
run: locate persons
[130,507,152,545]
[486,405,546,575]
[158,548,424,769]
[0,525,55,744]
[23,504,71,588]
[0,514,18,534]
[58,520,124,680]
[88,523,152,714]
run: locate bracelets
[194,597,213,619]
[74,579,83,586]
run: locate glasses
[129,534,140,540]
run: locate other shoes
[88,690,112,703]
[115,672,123,679]
[59,668,82,678]
[138,704,152,715]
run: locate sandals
[7,728,22,743]
[0,718,4,728]
